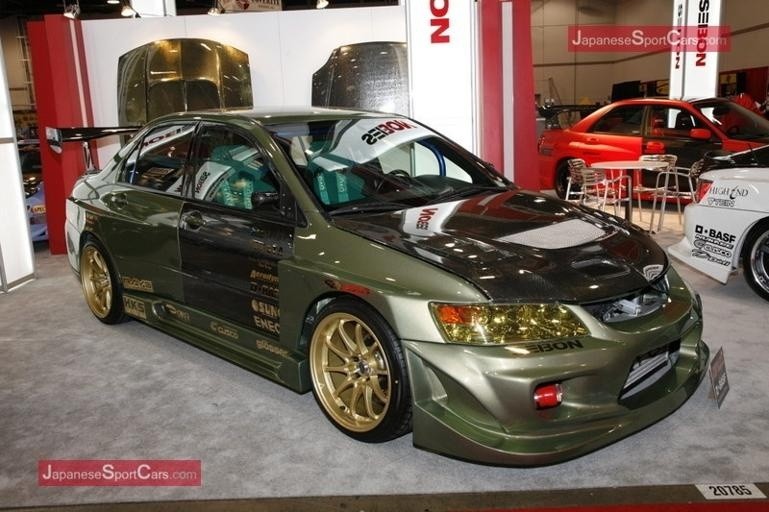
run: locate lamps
[61,0,331,17]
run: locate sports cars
[43,108,712,468]
[13,135,48,240]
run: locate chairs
[564,153,705,236]
